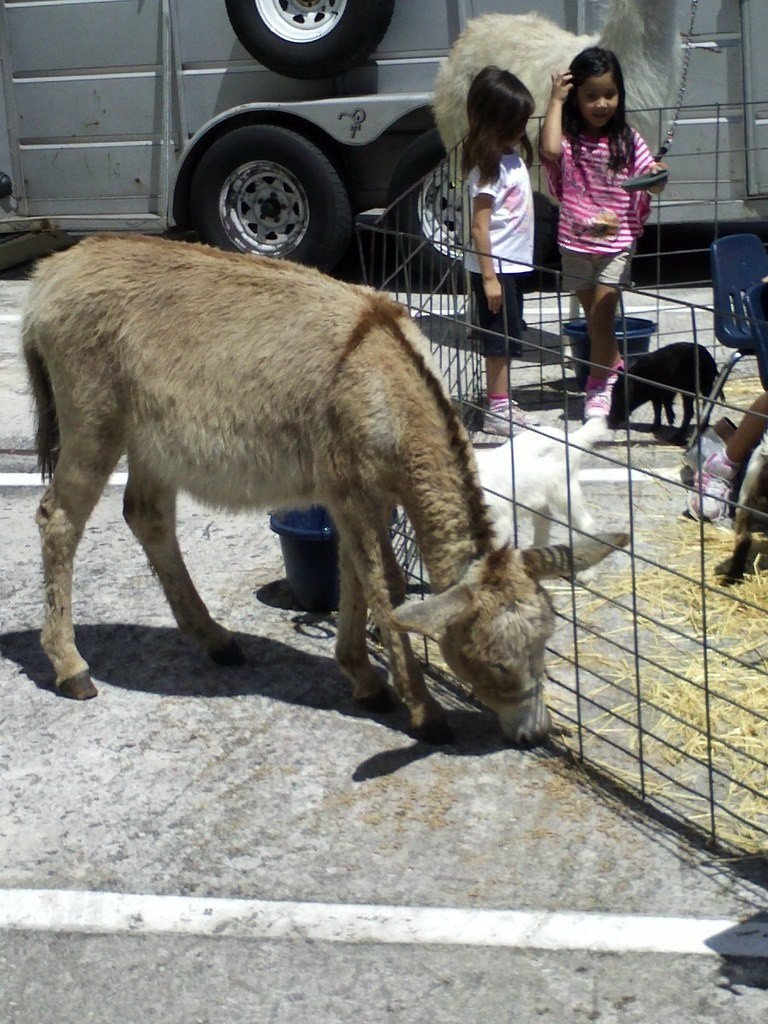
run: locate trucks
[0,0,768,278]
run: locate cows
[19,229,631,746]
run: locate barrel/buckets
[269,504,340,611]
[562,318,656,390]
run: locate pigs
[608,342,728,444]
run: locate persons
[458,65,541,440]
[686,389,768,526]
[536,46,670,423]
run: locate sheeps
[476,416,607,551]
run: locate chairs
[744,283,767,394]
[682,232,768,455]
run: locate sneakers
[692,452,738,522]
[583,374,620,418]
[481,400,541,438]
